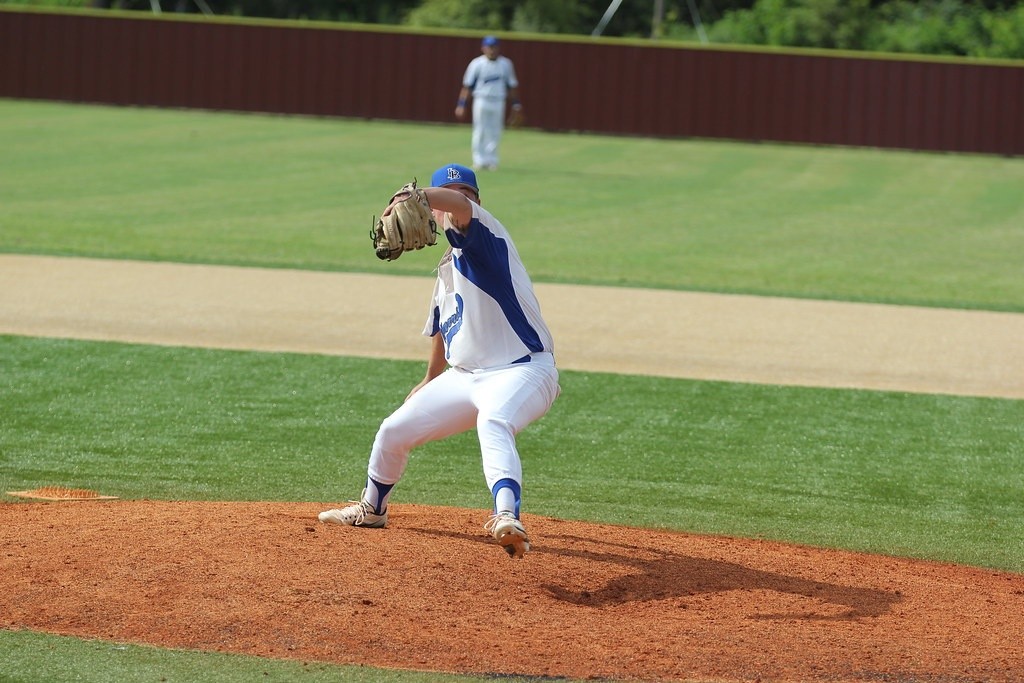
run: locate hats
[482,37,499,46]
[430,163,479,194]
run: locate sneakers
[483,511,528,557]
[318,496,386,528]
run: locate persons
[317,162,554,558]
[455,36,525,174]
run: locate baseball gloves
[369,174,442,263]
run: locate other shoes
[470,162,484,172]
[487,163,498,172]
